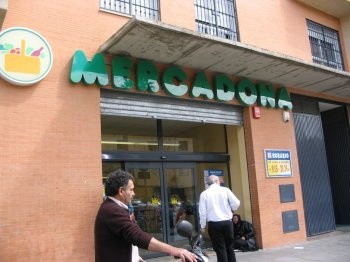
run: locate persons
[199,175,240,262]
[232,214,257,252]
[128,202,139,262]
[94,169,199,262]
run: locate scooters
[174,220,209,262]
